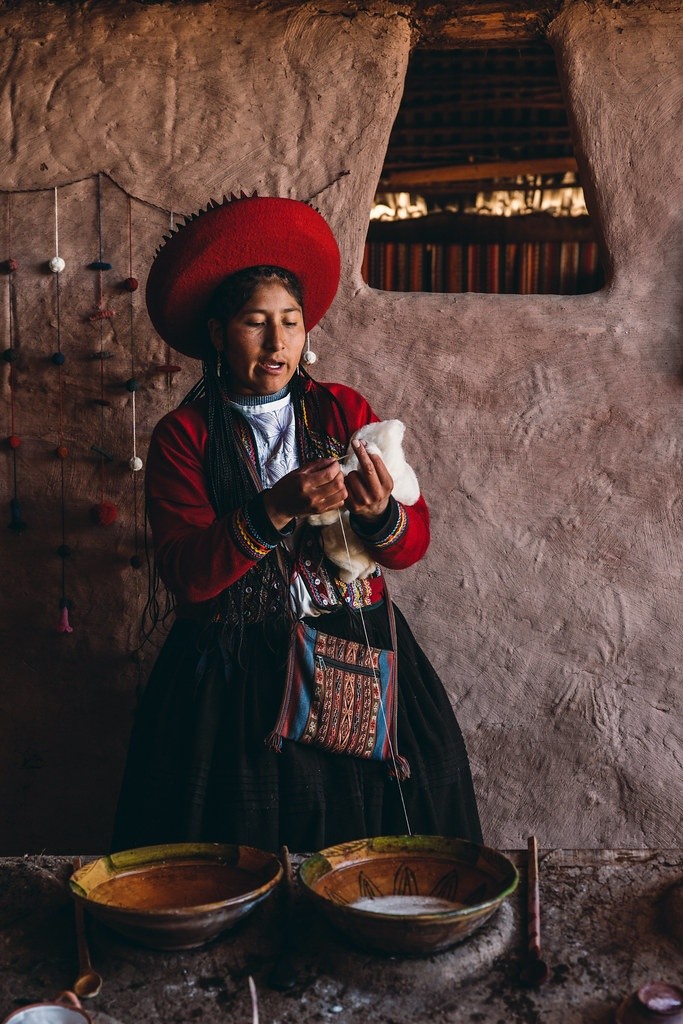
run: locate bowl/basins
[298,835,520,955]
[69,843,284,949]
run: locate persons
[141,190,460,841]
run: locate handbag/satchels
[263,611,412,780]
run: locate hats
[144,191,342,361]
